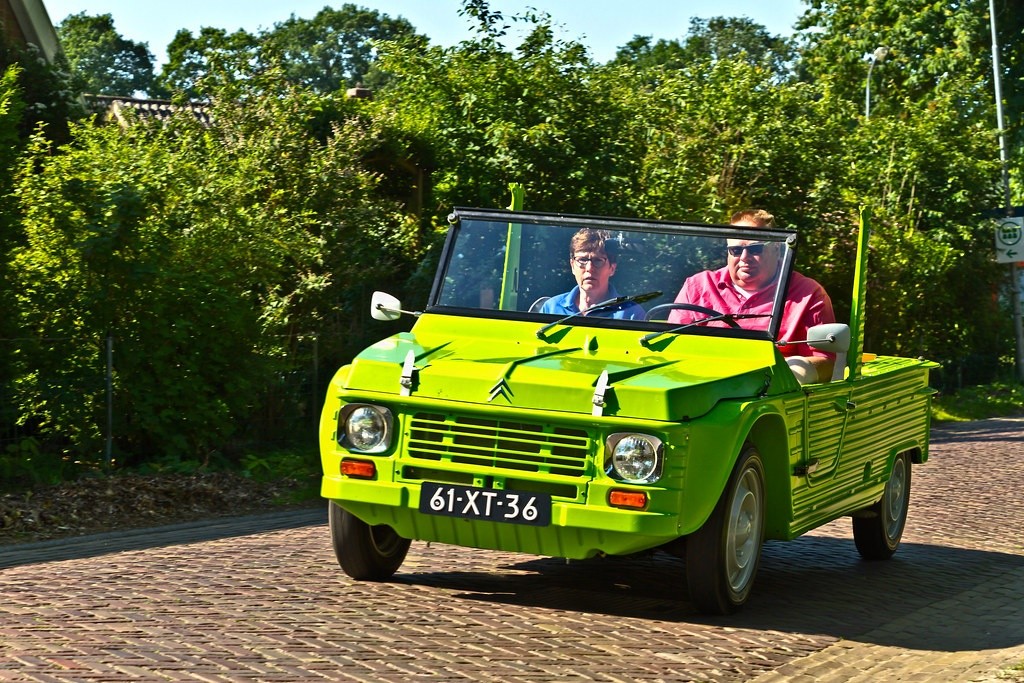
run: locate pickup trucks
[317,181,941,617]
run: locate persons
[668,208,837,385]
[539,231,648,322]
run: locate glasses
[573,256,608,269]
[727,241,771,258]
